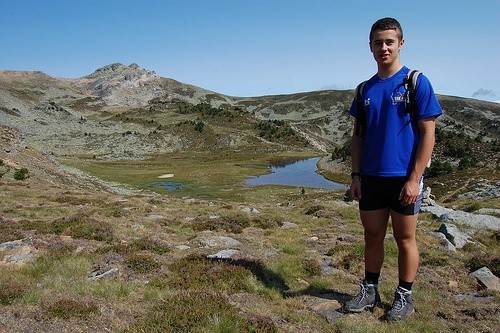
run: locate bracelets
[351,172,362,179]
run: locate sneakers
[345,279,381,312]
[384,286,414,321]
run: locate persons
[343,18,443,323]
